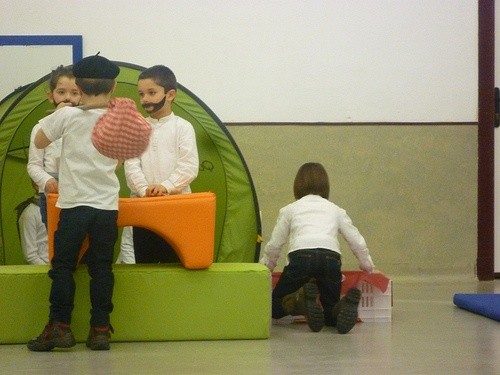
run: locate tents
[0,61,260,263]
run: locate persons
[260,163,374,334]
[116,226,136,265]
[125,66,200,197]
[17,180,48,265]
[27,66,82,229]
[27,56,121,353]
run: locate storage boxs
[272,272,391,321]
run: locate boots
[331,287,361,334]
[280,284,326,333]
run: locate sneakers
[87,324,111,350]
[27,323,77,352]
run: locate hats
[72,50,120,79]
